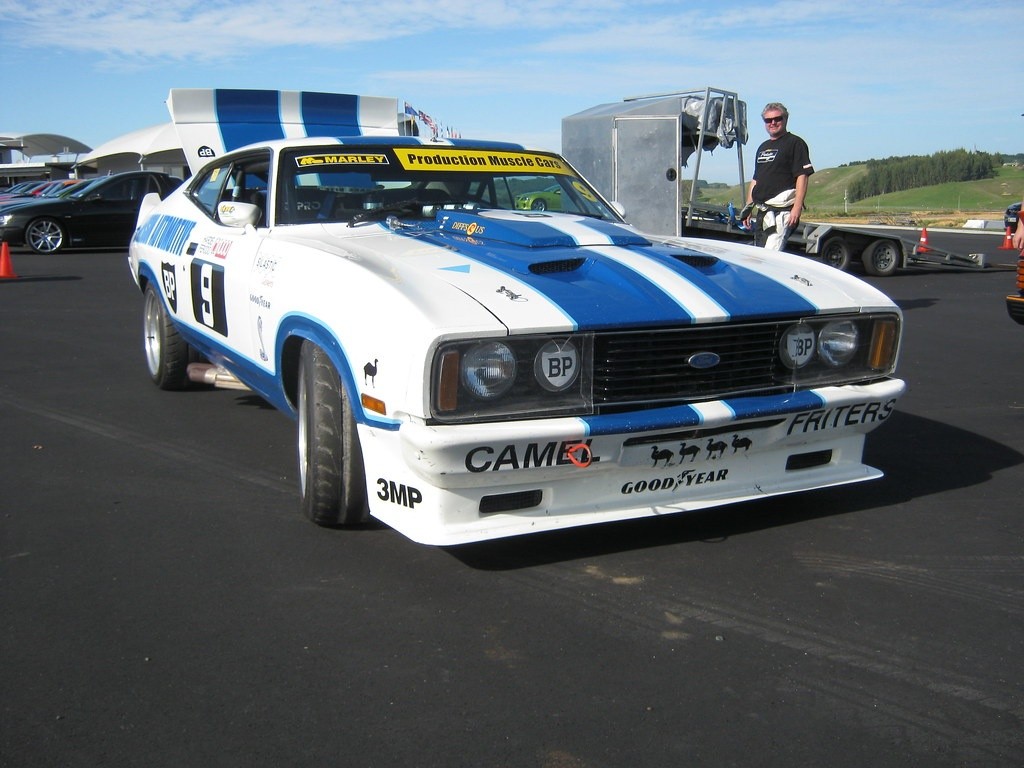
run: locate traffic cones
[0,242,19,278]
[916,227,932,255]
[998,226,1017,250]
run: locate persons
[1012,200,1024,252]
[743,102,814,254]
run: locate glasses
[764,116,784,123]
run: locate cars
[0,171,184,256]
[0,176,108,219]
[126,89,905,548]
[1003,201,1022,233]
